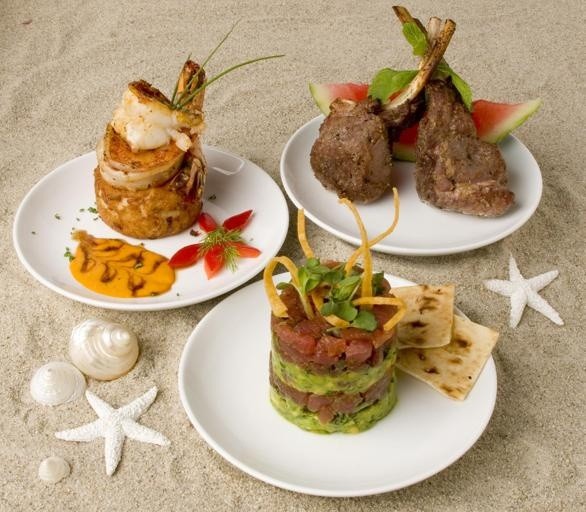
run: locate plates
[280,112,543,256]
[177,268,498,498]
[10,142,291,312]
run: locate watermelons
[307,82,541,162]
